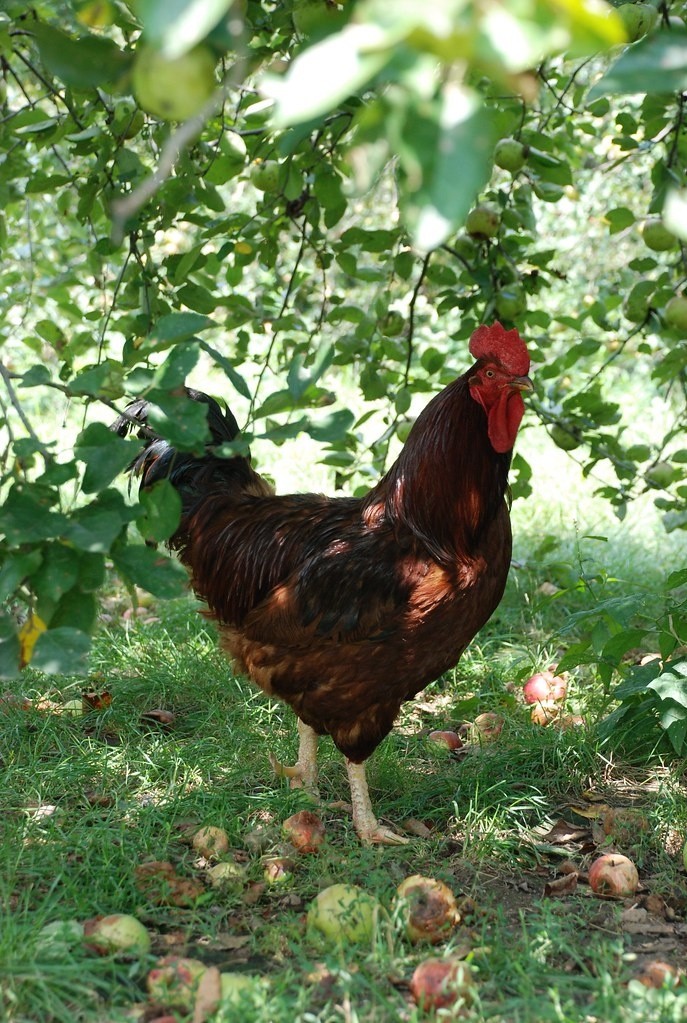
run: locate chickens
[111,321,546,840]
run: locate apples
[0,0,687,1018]
